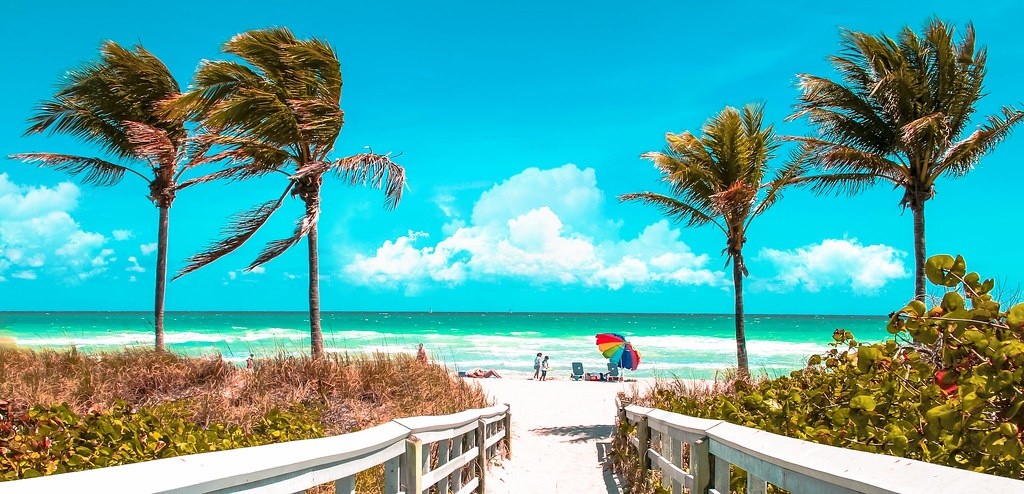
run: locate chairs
[607,362,620,382]
[572,362,585,381]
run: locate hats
[545,356,549,359]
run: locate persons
[533,353,549,381]
[467,368,502,379]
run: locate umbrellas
[595,333,641,371]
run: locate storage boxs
[458,372,466,377]
